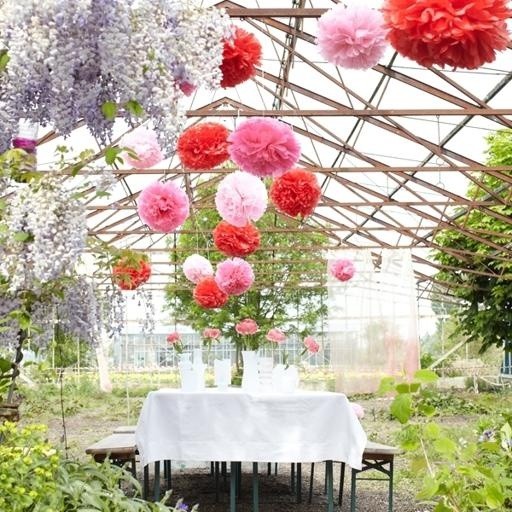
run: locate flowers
[167,319,318,365]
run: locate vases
[177,350,299,387]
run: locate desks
[134,387,367,512]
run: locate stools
[339,443,396,512]
[86,425,138,467]
